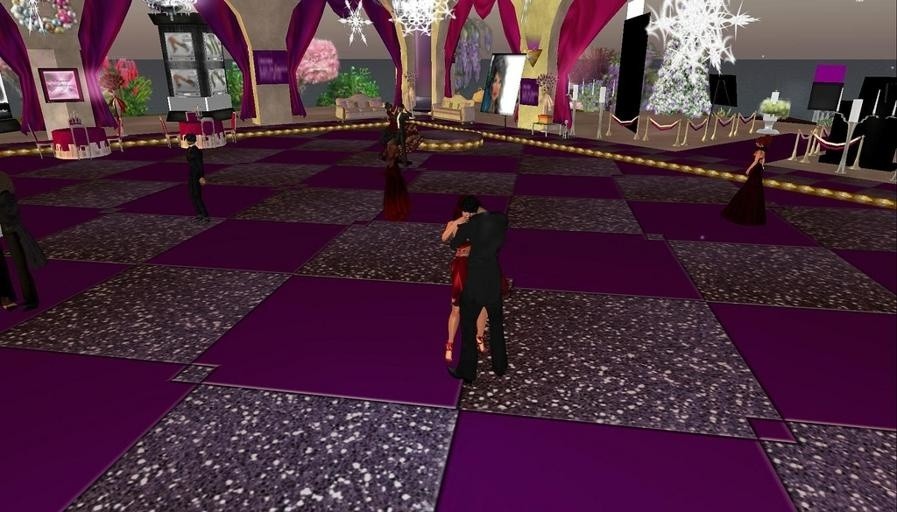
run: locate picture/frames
[37,68,84,105]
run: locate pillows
[340,99,378,109]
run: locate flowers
[758,98,790,120]
[98,74,128,98]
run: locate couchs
[335,93,390,123]
[431,94,475,126]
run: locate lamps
[141,1,198,22]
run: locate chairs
[26,117,126,160]
[158,111,238,149]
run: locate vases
[755,113,780,135]
